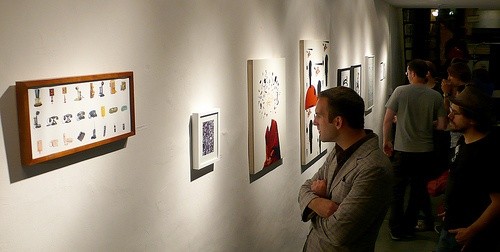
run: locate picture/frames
[365,55,376,111]
[380,62,384,80]
[351,65,361,96]
[337,68,353,89]
[15,71,135,169]
[192,108,221,171]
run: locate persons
[435,85,500,252]
[298,87,394,252]
[381,60,487,241]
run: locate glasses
[449,108,462,115]
[405,71,410,75]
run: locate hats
[448,87,494,117]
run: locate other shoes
[389,231,416,241]
[415,220,426,231]
[434,225,443,234]
